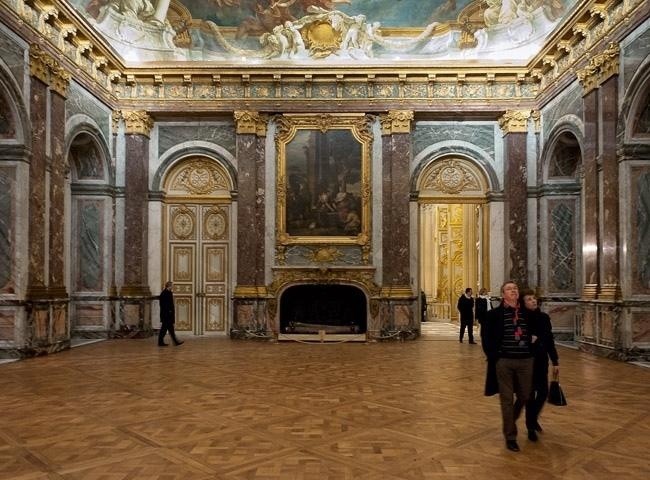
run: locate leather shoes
[175,341,184,346]
[469,341,477,344]
[159,343,169,346]
[536,420,543,432]
[506,439,520,452]
[527,428,538,442]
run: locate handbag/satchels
[547,369,567,406]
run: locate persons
[481,280,535,452]
[521,288,559,441]
[475,288,494,324]
[157,282,185,346]
[457,287,477,344]
[421,290,427,322]
